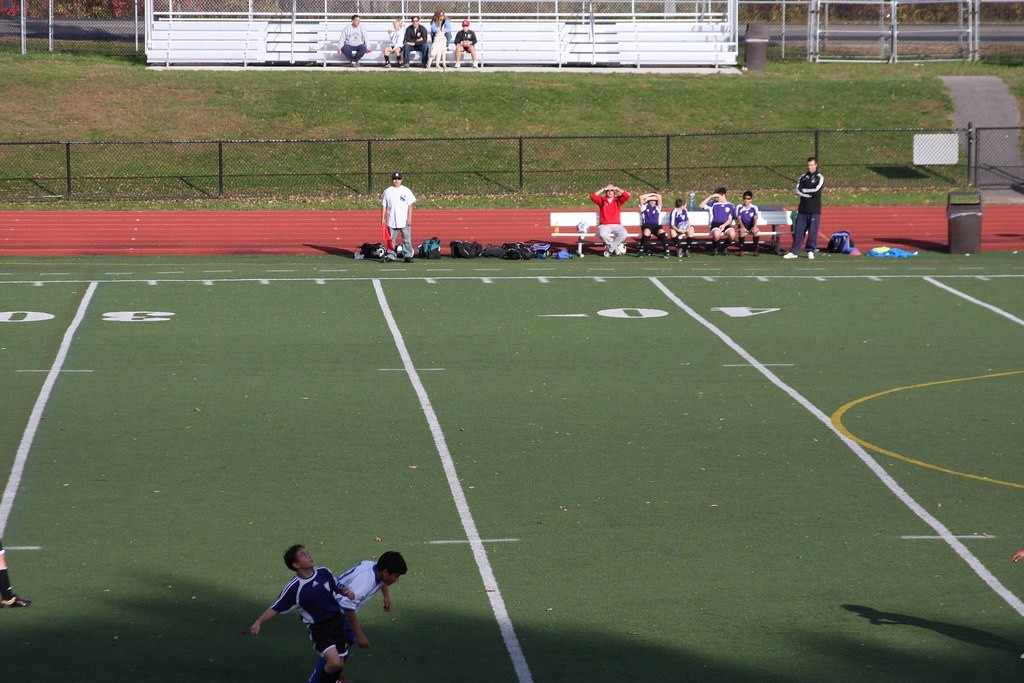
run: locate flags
[382,225,393,251]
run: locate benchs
[550,210,798,256]
[145,9,739,69]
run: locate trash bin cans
[945,189,985,254]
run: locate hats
[462,21,469,26]
[392,172,402,179]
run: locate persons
[638,191,670,258]
[338,15,372,67]
[699,187,736,256]
[590,184,631,258]
[669,198,694,258]
[381,172,416,263]
[250,544,409,683]
[736,191,759,256]
[0,540,32,608]
[783,157,825,259]
[384,9,478,69]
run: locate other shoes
[739,250,745,256]
[405,257,413,263]
[722,247,729,256]
[638,250,646,257]
[807,251,815,259]
[712,247,719,256]
[754,249,759,256]
[604,246,612,257]
[783,252,799,259]
[676,248,691,257]
[662,250,671,258]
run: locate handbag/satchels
[360,242,386,259]
[828,230,854,254]
[418,237,442,259]
[450,238,573,260]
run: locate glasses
[743,197,751,201]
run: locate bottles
[554,222,560,233]
[689,191,694,207]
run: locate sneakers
[1,597,32,608]
